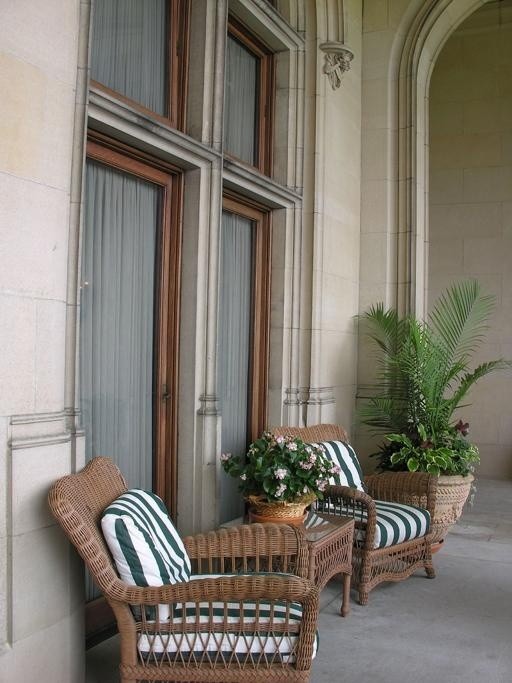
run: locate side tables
[219,512,355,616]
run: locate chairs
[44,454,321,681]
[264,422,438,603]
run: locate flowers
[221,432,343,507]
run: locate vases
[246,498,311,535]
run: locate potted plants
[348,276,512,550]
